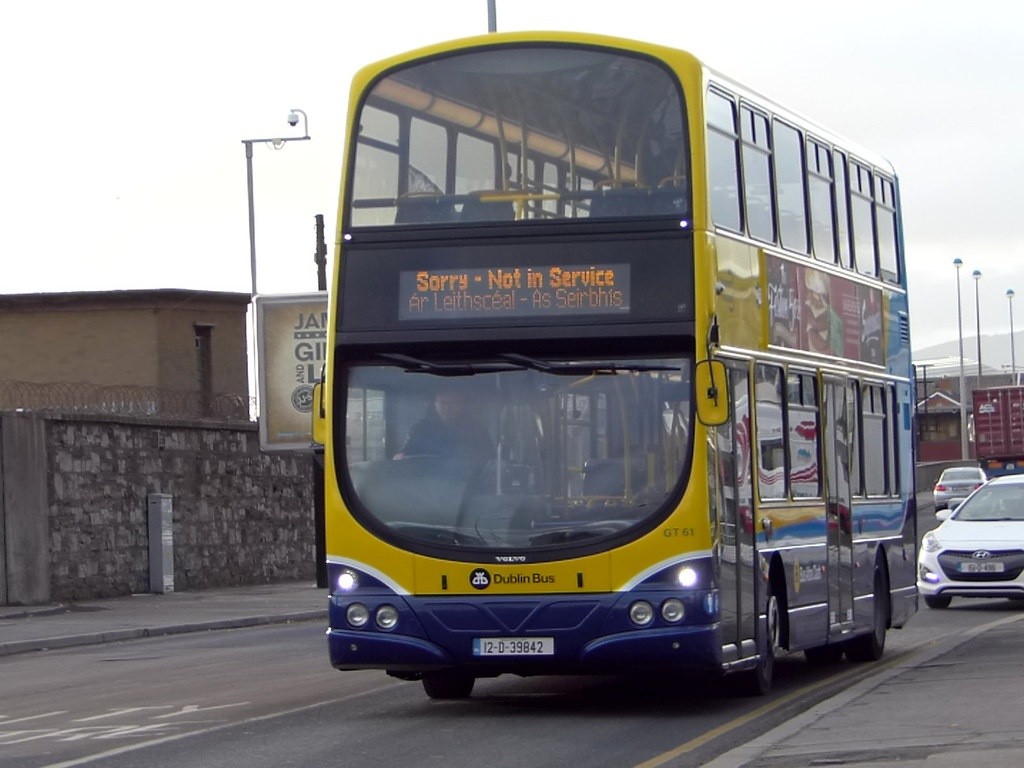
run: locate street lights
[1006,288,1016,386]
[242,108,311,299]
[952,258,971,465]
[971,271,985,383]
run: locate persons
[391,385,499,463]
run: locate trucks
[969,386,1024,474]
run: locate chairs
[394,171,687,224]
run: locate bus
[318,29,917,708]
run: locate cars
[932,465,987,510]
[911,474,1024,607]
